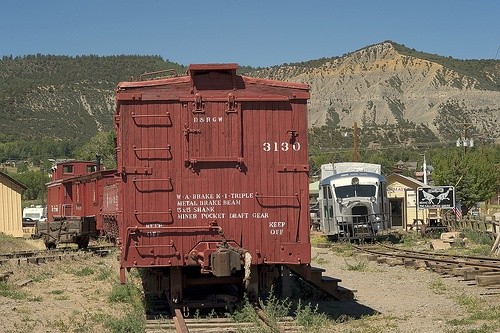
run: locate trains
[41,62,312,312]
[318,162,388,244]
